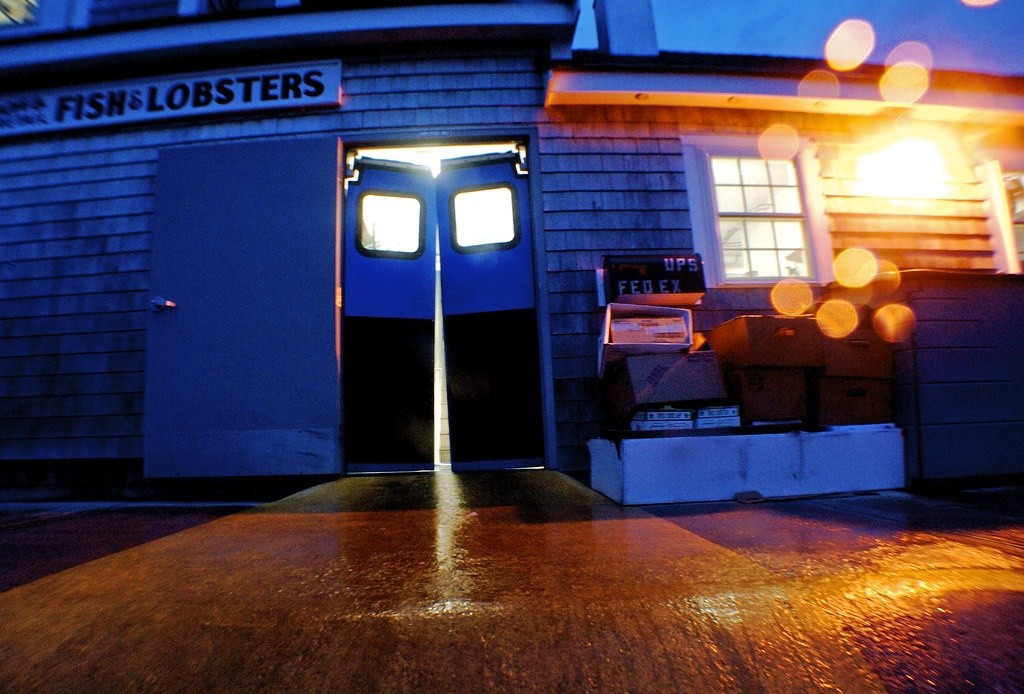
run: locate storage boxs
[825,330,893,379]
[587,424,903,507]
[622,349,727,418]
[820,379,893,425]
[706,314,825,368]
[596,303,694,376]
[597,263,707,306]
[730,369,808,423]
